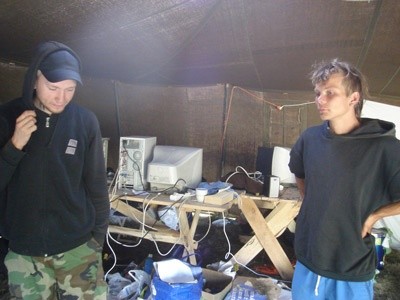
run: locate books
[204,190,234,206]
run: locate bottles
[144,254,153,273]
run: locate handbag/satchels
[140,253,204,300]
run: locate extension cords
[170,189,195,202]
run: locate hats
[39,50,83,87]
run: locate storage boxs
[201,268,233,300]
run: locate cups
[196,187,208,202]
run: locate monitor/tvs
[271,146,296,184]
[147,145,203,193]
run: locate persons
[0,40,111,300]
[288,58,400,300]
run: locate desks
[106,185,247,265]
[230,187,303,281]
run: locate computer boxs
[118,136,157,193]
